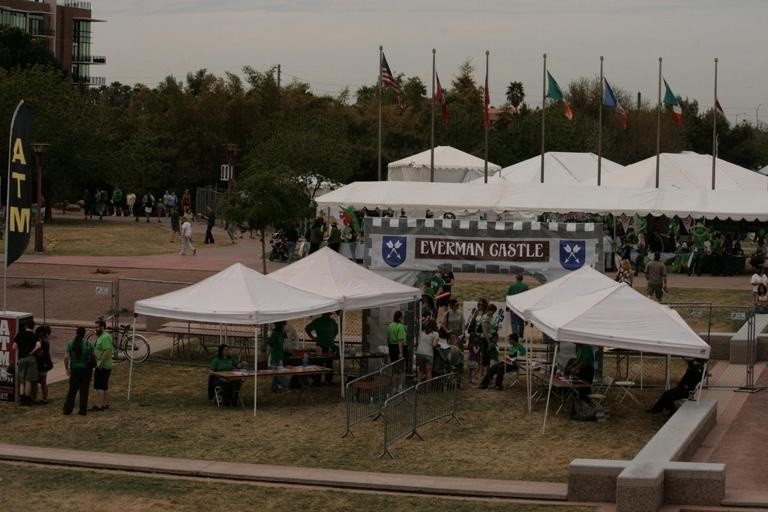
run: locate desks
[511,357,591,413]
[156,321,263,361]
[211,349,402,406]
[268,330,363,353]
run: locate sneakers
[19,399,48,406]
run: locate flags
[662,78,682,126]
[483,78,491,129]
[602,76,628,130]
[434,72,449,125]
[380,52,407,111]
[545,70,574,120]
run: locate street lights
[27,142,52,250]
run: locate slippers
[88,405,104,412]
[101,405,109,409]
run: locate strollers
[431,341,469,391]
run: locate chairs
[585,375,613,416]
[614,364,637,404]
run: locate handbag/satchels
[84,343,96,368]
[35,353,52,373]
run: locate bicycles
[84,313,153,364]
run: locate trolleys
[349,356,401,405]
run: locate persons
[88,320,113,413]
[29,325,53,404]
[568,343,598,422]
[12,319,41,405]
[265,270,529,394]
[209,344,248,411]
[644,356,707,418]
[62,326,97,416]
[598,215,768,314]
[82,184,238,257]
[280,216,359,264]
